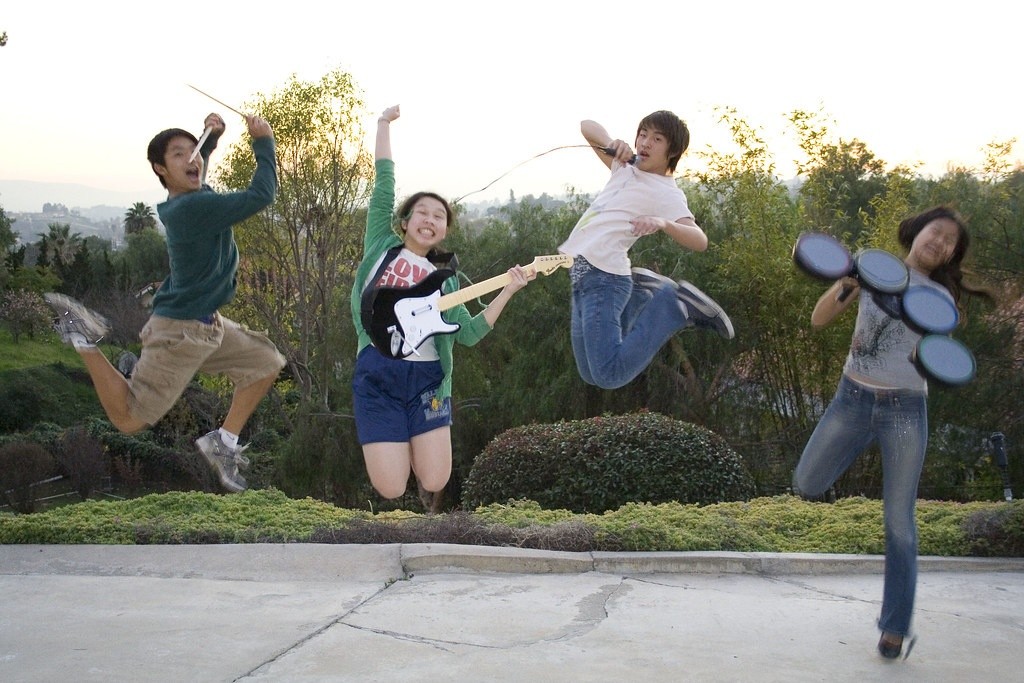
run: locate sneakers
[632,266,679,293]
[196,432,247,491]
[44,293,112,343]
[675,281,736,339]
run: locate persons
[795,202,972,661]
[352,105,537,500]
[554,109,737,394]
[43,112,289,492]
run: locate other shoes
[878,633,903,658]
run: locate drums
[788,229,853,279]
[855,248,912,294]
[912,332,978,388]
[899,282,961,334]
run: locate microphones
[603,148,640,168]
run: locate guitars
[360,254,574,361]
